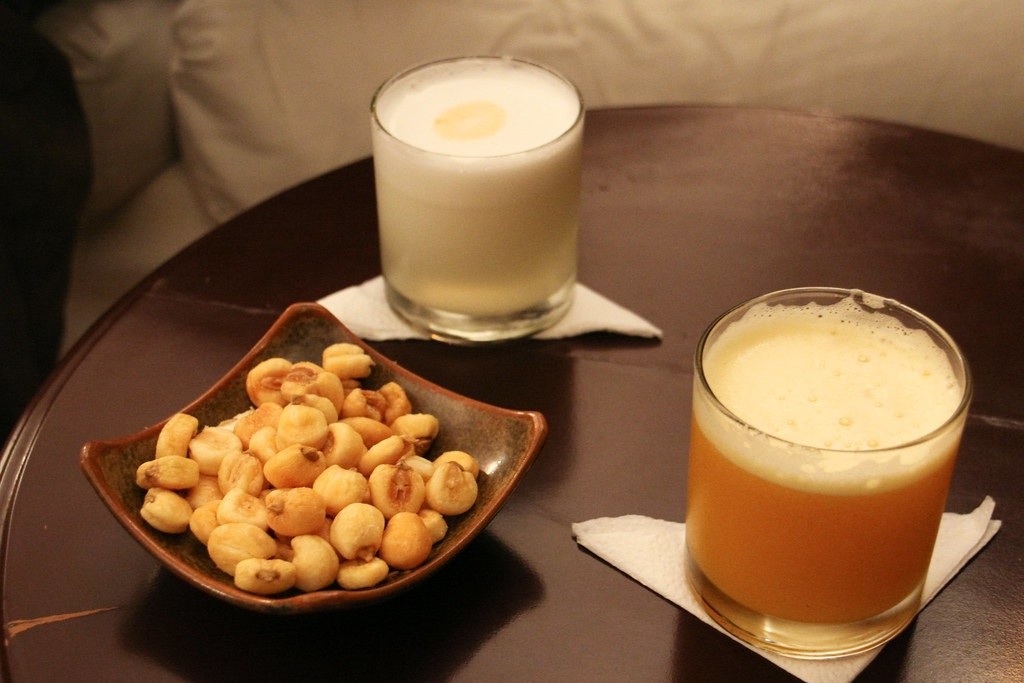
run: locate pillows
[165,0,785,224]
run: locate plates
[82,300,548,616]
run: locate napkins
[317,272,664,340]
[571,494,1005,683]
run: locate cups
[683,286,975,661]
[369,54,584,340]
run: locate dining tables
[1,97,1021,683]
